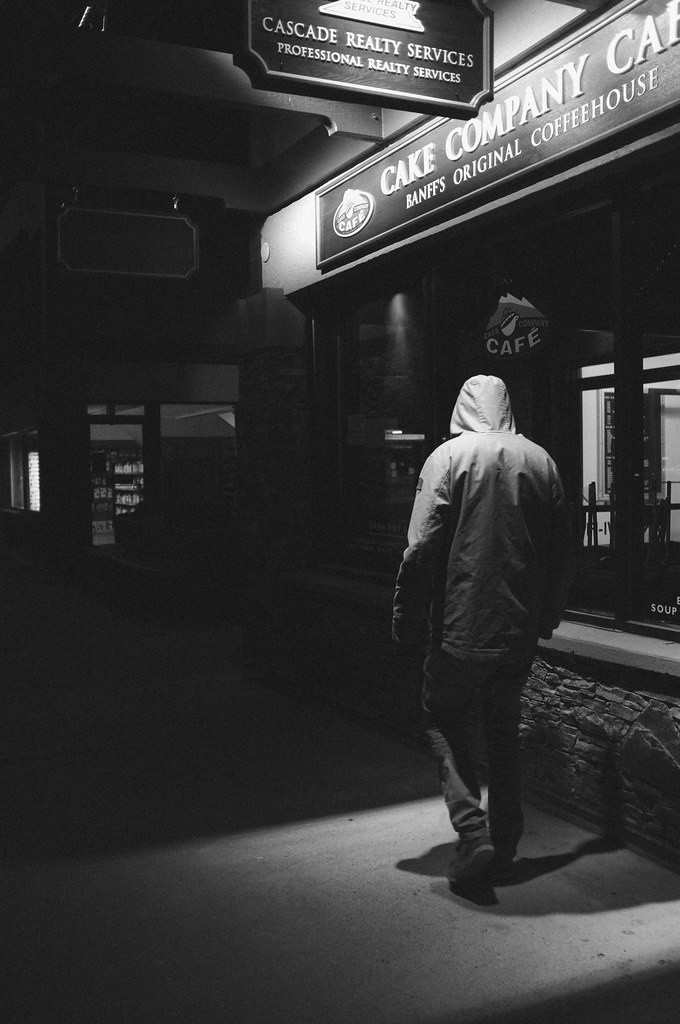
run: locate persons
[390,372,574,887]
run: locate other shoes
[446,836,513,888]
